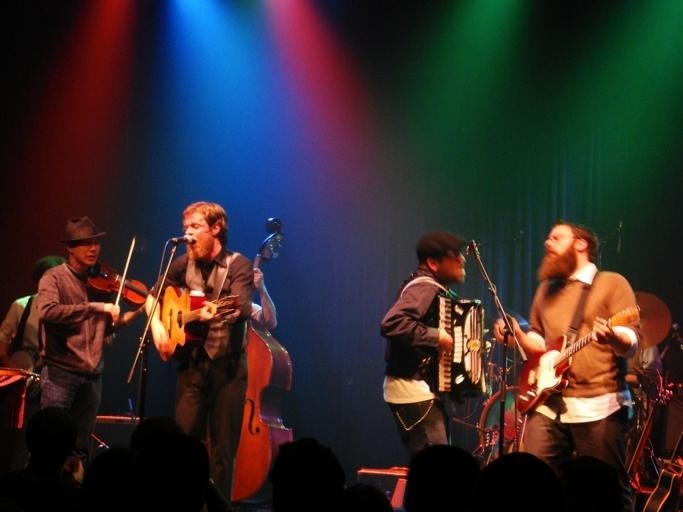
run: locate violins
[85,259,148,306]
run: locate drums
[476,385,526,464]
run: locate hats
[417,232,466,257]
[57,216,106,242]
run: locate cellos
[230,217,293,503]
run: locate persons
[0,256,68,376]
[249,268,277,332]
[624,347,664,395]
[1,404,563,512]
[380,231,492,455]
[23,215,145,462]
[660,322,683,392]
[145,201,254,509]
[493,221,643,512]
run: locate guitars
[161,285,242,357]
[643,433,683,511]
[516,304,641,414]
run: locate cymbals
[633,292,673,349]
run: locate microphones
[169,234,198,247]
[462,239,485,255]
